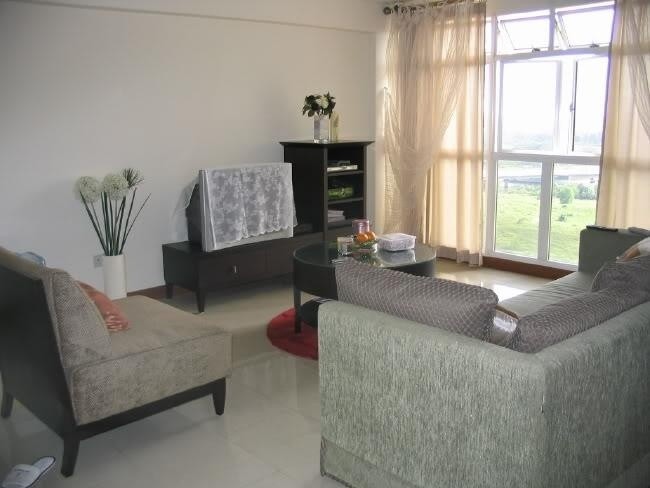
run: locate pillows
[331,259,496,343]
[507,286,650,355]
[592,254,650,291]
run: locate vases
[99,255,127,301]
[314,114,330,142]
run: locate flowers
[302,91,337,119]
[74,167,152,255]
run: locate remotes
[586,225,618,232]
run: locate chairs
[0,248,231,478]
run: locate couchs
[315,230,650,487]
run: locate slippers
[1,454,57,488]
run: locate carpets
[268,306,318,360]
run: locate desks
[162,226,325,313]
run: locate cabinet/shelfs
[280,139,376,239]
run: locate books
[328,216,345,223]
[328,210,344,217]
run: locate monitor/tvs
[186,163,294,252]
